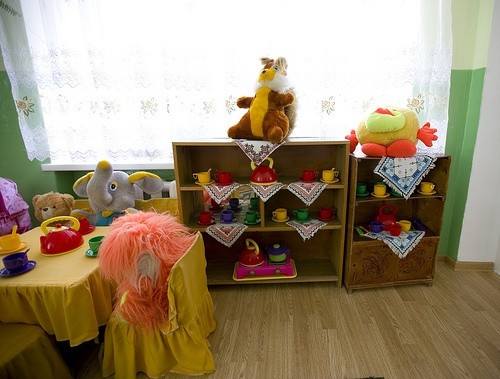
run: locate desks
[0,225,125,347]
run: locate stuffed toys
[346,105,439,157]
[227,56,298,143]
[96,208,199,328]
[31,191,73,221]
[70,160,164,225]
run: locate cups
[89,235,104,252]
[193,171,232,183]
[199,197,259,222]
[0,234,21,249]
[2,252,28,272]
[302,170,339,181]
[369,220,412,236]
[272,208,337,219]
[358,178,435,195]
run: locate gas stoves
[236,250,295,279]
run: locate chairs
[0,200,216,379]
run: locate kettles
[234,238,264,268]
[40,215,85,256]
[372,204,397,223]
[248,157,278,185]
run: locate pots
[267,244,288,262]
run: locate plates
[243,218,261,225]
[294,215,311,222]
[356,190,436,197]
[85,249,98,258]
[0,241,28,254]
[220,218,238,224]
[193,179,339,187]
[272,216,290,223]
[197,218,216,226]
[317,216,335,221]
[0,261,36,277]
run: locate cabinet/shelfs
[170,141,350,288]
[342,154,451,293]
